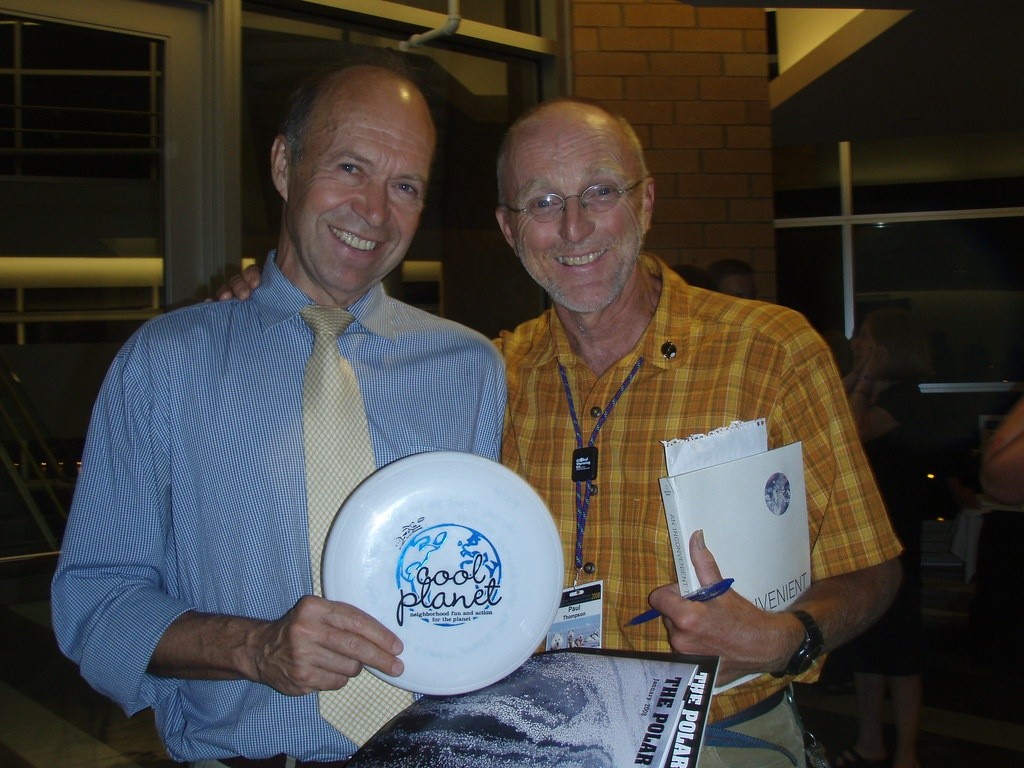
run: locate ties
[299,305,416,748]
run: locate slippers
[831,747,891,768]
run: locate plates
[321,452,564,694]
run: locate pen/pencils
[623,576,735,629]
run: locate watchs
[771,609,825,680]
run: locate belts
[704,687,797,767]
[216,752,352,768]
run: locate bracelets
[857,375,874,383]
[851,387,871,404]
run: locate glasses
[501,177,642,223]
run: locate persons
[206,95,903,768]
[48,60,506,768]
[666,254,1024,768]
